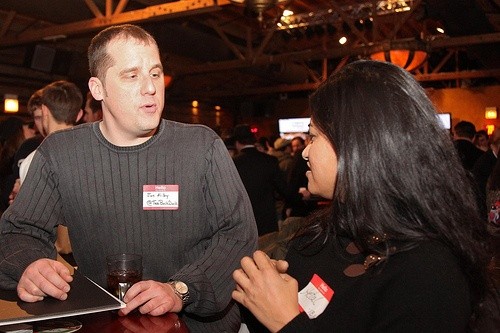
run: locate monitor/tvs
[277,116,311,139]
[436,112,452,130]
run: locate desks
[0,309,190,333]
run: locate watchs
[167,281,191,305]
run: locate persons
[231,60,500,333]
[222,135,318,232]
[448,127,500,231]
[0,25,258,333]
[0,81,103,270]
[446,120,496,225]
[232,126,310,237]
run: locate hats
[231,124,254,141]
[274,138,290,149]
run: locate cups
[106,254,142,316]
